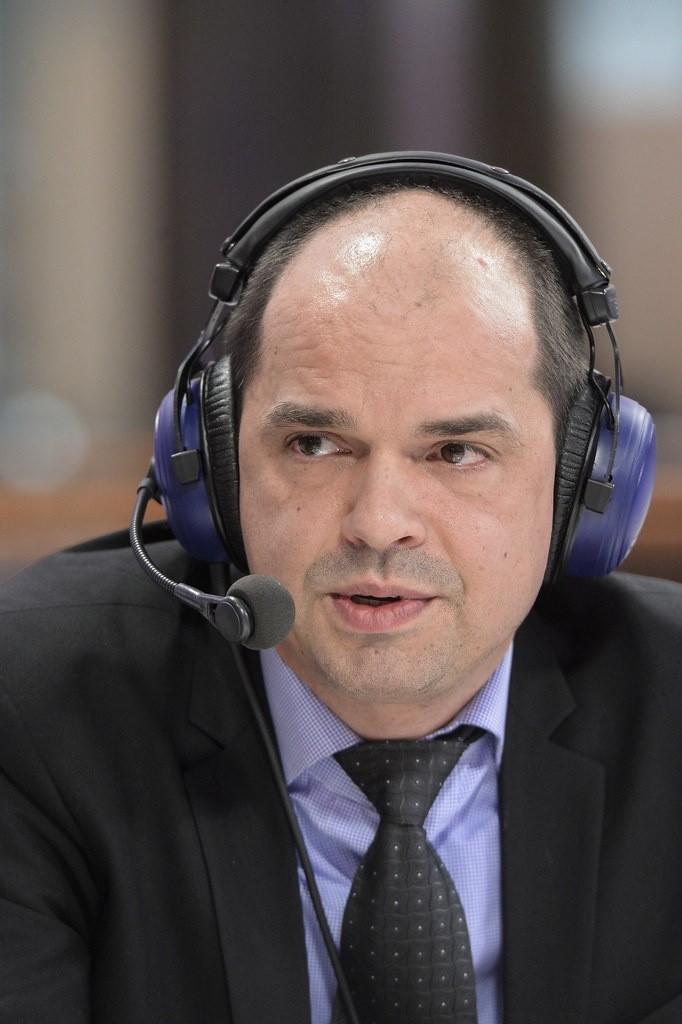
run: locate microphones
[124,476,297,648]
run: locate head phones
[152,149,655,585]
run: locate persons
[5,149,681,1023]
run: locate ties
[327,725,489,1024]
